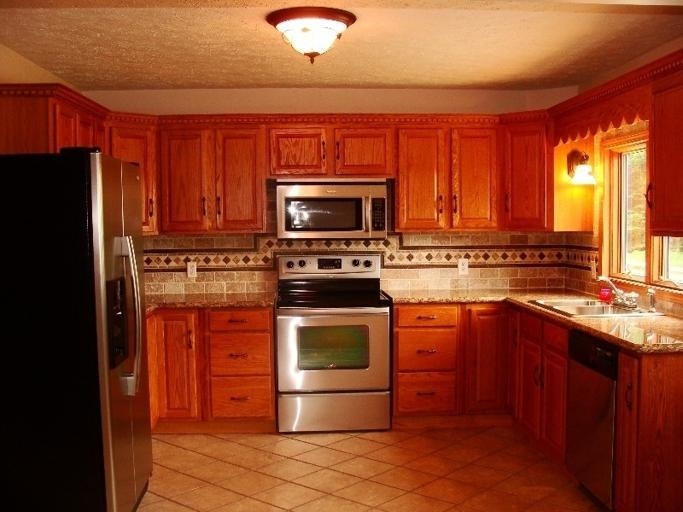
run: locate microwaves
[274,183,388,241]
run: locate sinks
[554,306,654,318]
[536,297,596,306]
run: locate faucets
[598,276,636,310]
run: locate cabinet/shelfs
[1,82,108,155]
[145,309,277,436]
[511,309,571,466]
[648,48,683,239]
[160,112,267,236]
[392,303,511,429]
[107,111,160,238]
[498,109,594,232]
[396,113,498,231]
[614,352,683,511]
[267,114,395,179]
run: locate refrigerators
[0,146,153,512]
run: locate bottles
[600,289,611,302]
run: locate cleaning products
[599,281,614,302]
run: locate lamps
[568,150,597,186]
[263,5,358,66]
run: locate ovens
[275,306,392,434]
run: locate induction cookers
[275,282,393,308]
